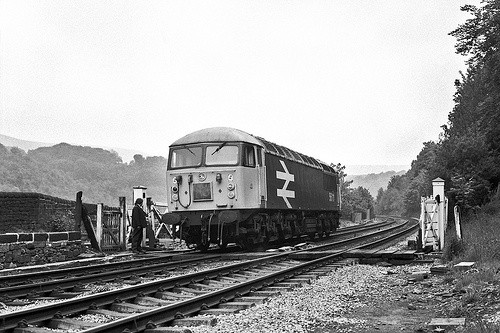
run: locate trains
[161,127,341,253]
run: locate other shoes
[133,248,139,253]
[137,248,144,252]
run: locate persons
[131,198,148,254]
